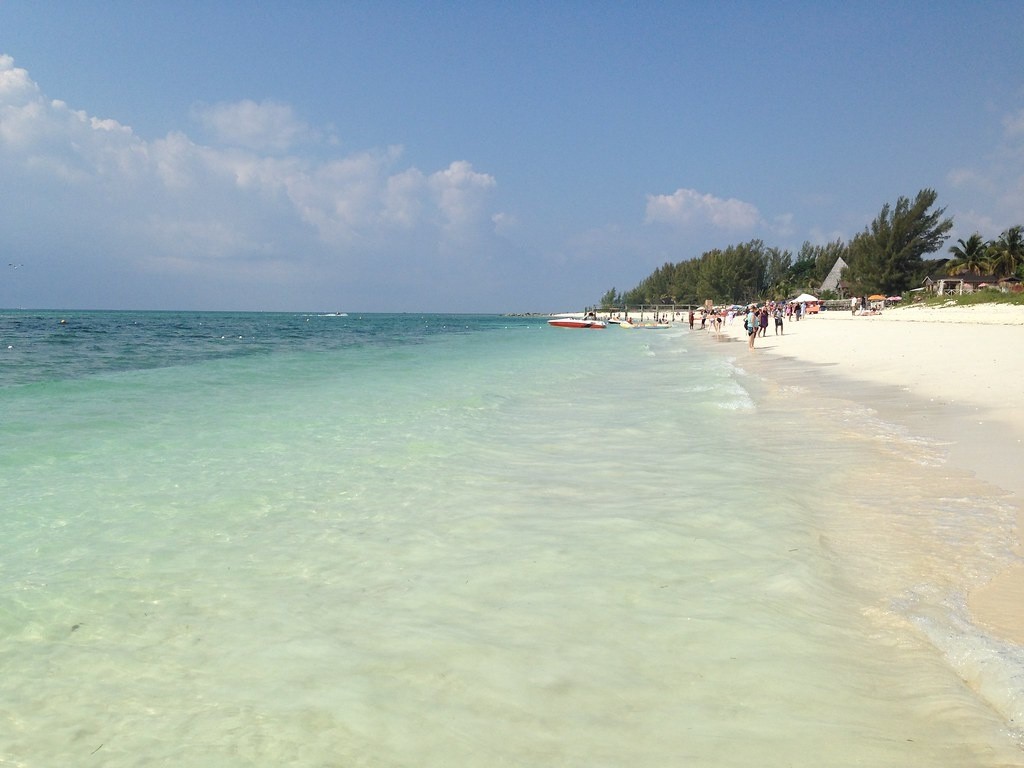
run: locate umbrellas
[868,295,886,300]
[978,283,988,288]
[886,296,902,305]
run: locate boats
[608,317,626,324]
[546,311,606,329]
[620,321,672,330]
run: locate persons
[851,296,858,316]
[689,300,806,350]
